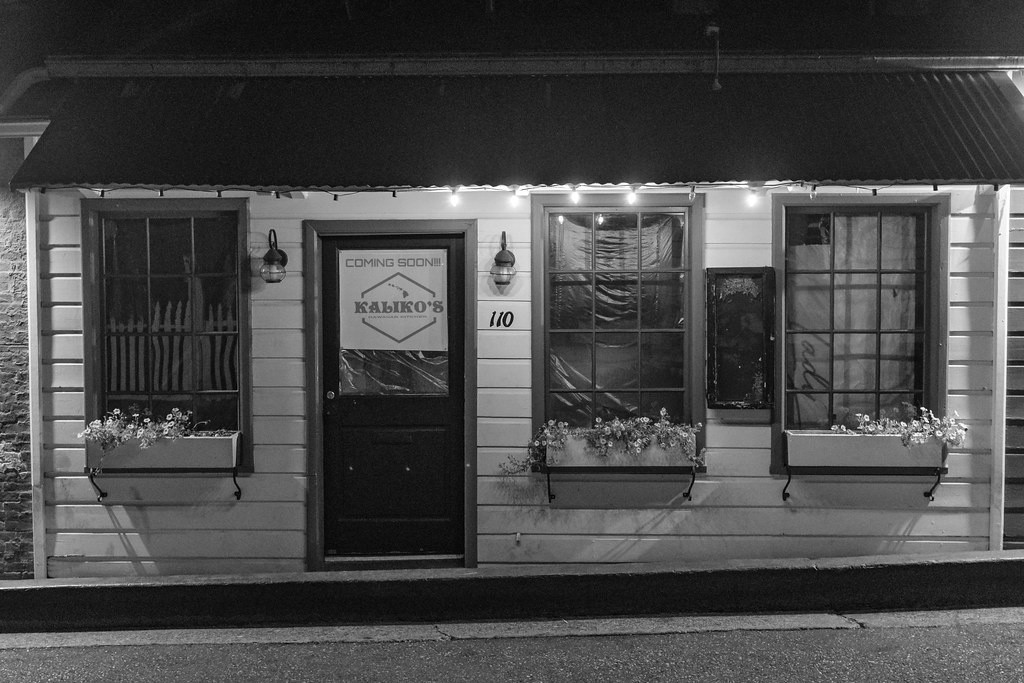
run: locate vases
[785,428,945,468]
[547,429,699,467]
[84,429,242,469]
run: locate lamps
[260,229,289,284]
[489,231,516,288]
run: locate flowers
[75,404,210,450]
[494,404,713,476]
[835,401,968,449]
